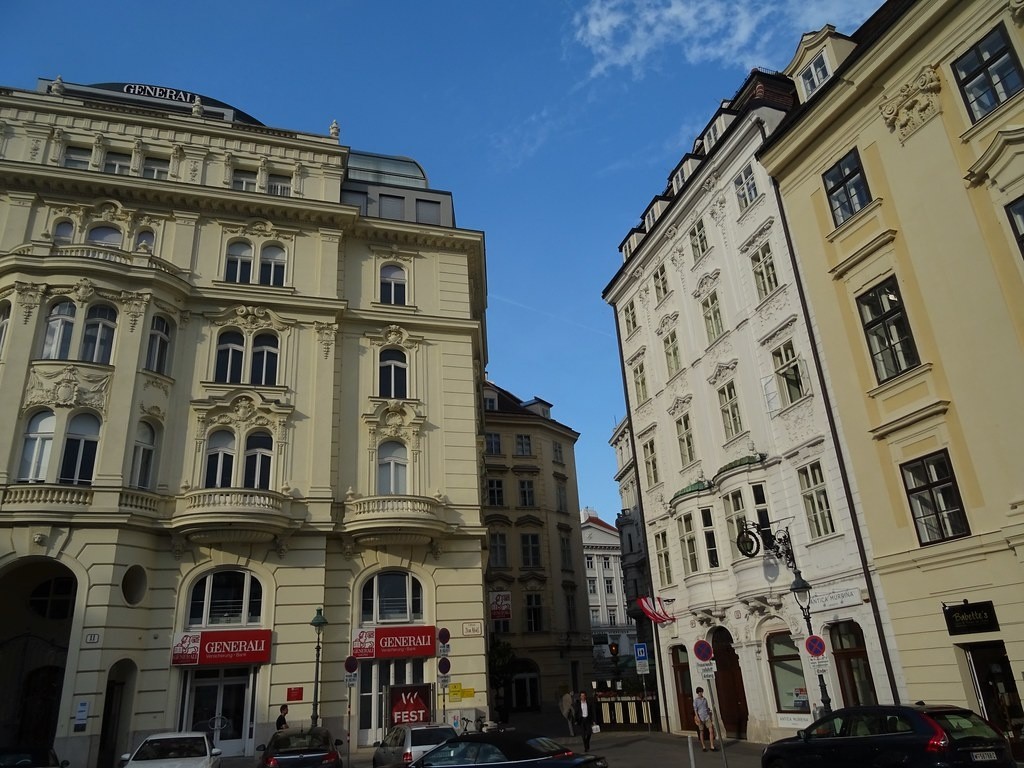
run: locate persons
[573,691,596,752]
[693,687,719,751]
[276,704,291,731]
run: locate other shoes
[702,748,707,752]
[710,747,718,751]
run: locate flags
[638,597,672,623]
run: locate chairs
[850,721,870,735]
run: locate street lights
[609,642,621,691]
[309,607,327,728]
[790,565,837,736]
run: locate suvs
[372,721,458,768]
[761,702,1017,768]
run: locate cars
[120,732,222,768]
[391,731,608,768]
[0,735,70,768]
[256,726,344,768]
[192,720,234,740]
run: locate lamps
[736,514,797,570]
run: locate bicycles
[460,715,505,735]
